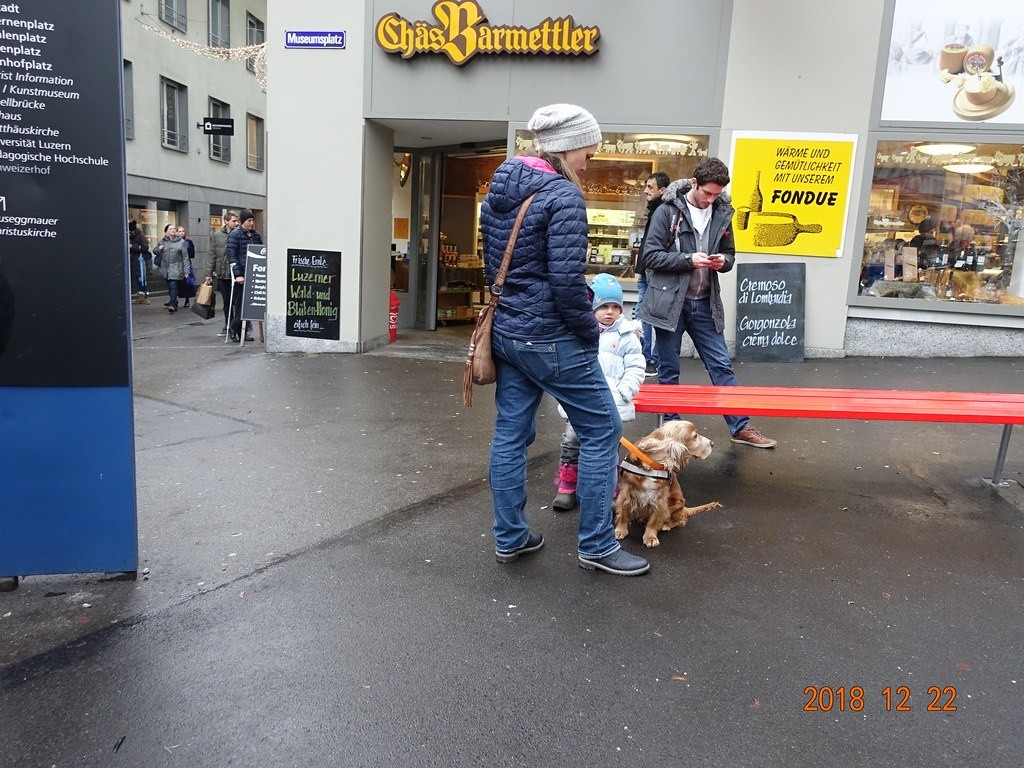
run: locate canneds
[441,244,457,261]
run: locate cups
[459,255,483,268]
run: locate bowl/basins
[437,310,445,317]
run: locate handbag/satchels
[190,281,216,319]
[463,304,497,407]
[154,248,163,267]
[197,279,212,305]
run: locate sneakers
[730,424,778,447]
[645,361,658,377]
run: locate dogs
[612,419,720,548]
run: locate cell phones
[707,255,721,260]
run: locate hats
[592,274,624,314]
[129,220,137,231]
[918,218,937,233]
[240,209,254,224]
[527,104,602,154]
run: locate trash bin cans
[389,289,401,343]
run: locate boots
[131,292,145,304]
[610,478,621,508]
[552,463,577,509]
[142,291,151,305]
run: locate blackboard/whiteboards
[734,262,806,362]
[285,248,341,340]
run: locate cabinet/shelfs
[436,244,485,321]
[587,224,633,250]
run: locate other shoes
[168,306,178,312]
[164,300,178,306]
[183,302,190,307]
[216,327,231,336]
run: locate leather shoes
[495,531,544,563]
[578,547,650,576]
[228,325,239,343]
[238,331,254,341]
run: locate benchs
[630,382,1024,485]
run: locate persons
[894,238,910,253]
[945,224,979,271]
[552,272,647,511]
[205,212,254,337]
[227,210,263,342]
[128,220,149,291]
[153,223,195,312]
[634,173,669,376]
[910,219,939,268]
[480,102,650,575]
[642,156,778,448]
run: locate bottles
[589,249,631,265]
[588,225,630,235]
[440,232,449,245]
[445,280,482,290]
[952,237,1001,271]
[918,240,949,268]
[918,268,953,298]
[588,238,628,248]
[440,245,457,268]
